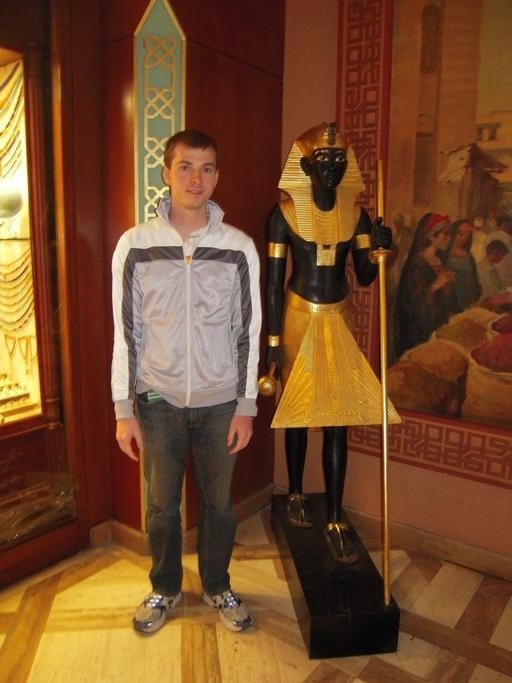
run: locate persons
[395,211,511,345]
[267,122,402,562]
[110,129,261,634]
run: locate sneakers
[203,589,253,631]
[132,590,183,633]
